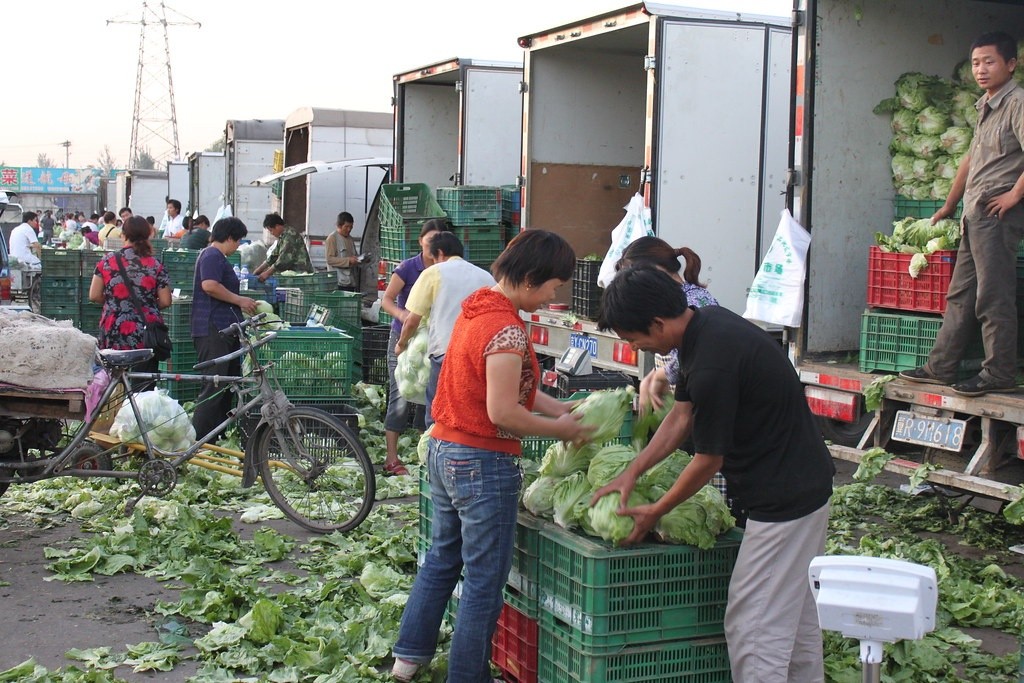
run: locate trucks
[245,104,393,329]
[91,149,228,237]
[761,1,1024,525]
[380,55,529,204]
[221,117,293,242]
[509,0,797,403]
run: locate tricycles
[0,310,376,536]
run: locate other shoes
[899,368,948,384]
[391,657,419,683]
[952,374,1015,396]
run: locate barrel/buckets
[0,277,11,300]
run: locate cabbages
[518,384,737,549]
[872,42,1024,276]
[820,375,1024,683]
[242,300,348,396]
[394,321,432,397]
[0,382,510,683]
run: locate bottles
[306,316,316,327]
[232,264,241,281]
[240,266,249,291]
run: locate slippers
[383,462,406,476]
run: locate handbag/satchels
[143,321,173,361]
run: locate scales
[809,555,938,683]
[51,238,62,248]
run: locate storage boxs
[42,238,363,459]
[858,195,1023,383]
[360,182,519,426]
[573,258,605,322]
[264,149,284,246]
[417,373,743,683]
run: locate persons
[9,211,42,270]
[325,212,370,292]
[382,219,454,477]
[189,217,262,446]
[613,237,721,415]
[595,264,835,683]
[89,215,173,467]
[33,199,214,252]
[394,231,500,431]
[898,31,1024,398]
[387,229,577,683]
[252,213,314,283]
